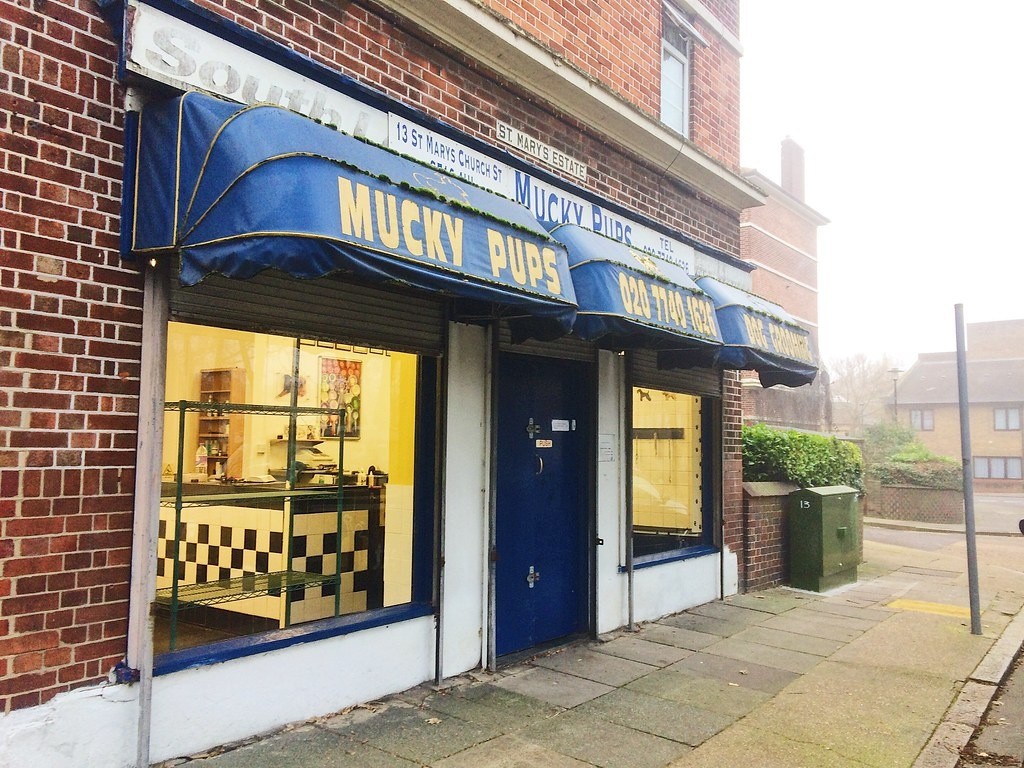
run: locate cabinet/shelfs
[155,400,344,652]
[195,367,247,478]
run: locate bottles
[206,438,226,484]
[195,442,208,482]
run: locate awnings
[118,87,580,342]
[656,274,817,390]
[507,220,725,372]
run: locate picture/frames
[317,356,363,440]
